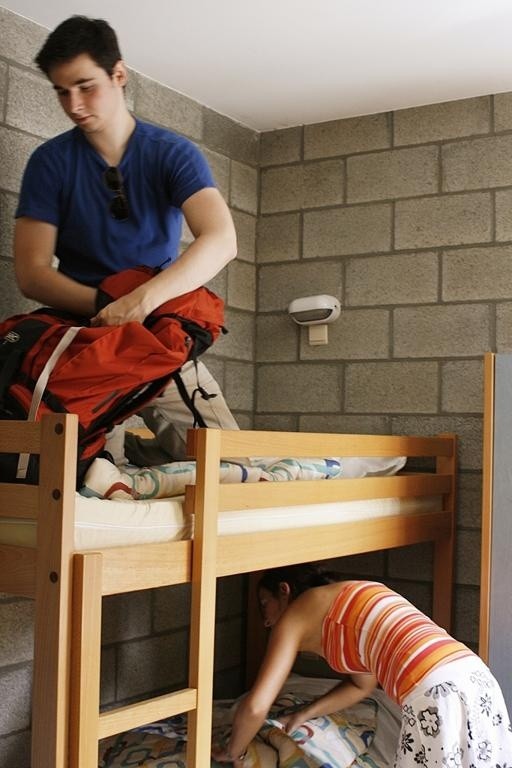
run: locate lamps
[289,294,341,327]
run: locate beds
[2,412,461,768]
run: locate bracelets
[221,741,250,761]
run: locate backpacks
[0,269,227,491]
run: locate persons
[212,564,512,768]
[13,13,252,468]
